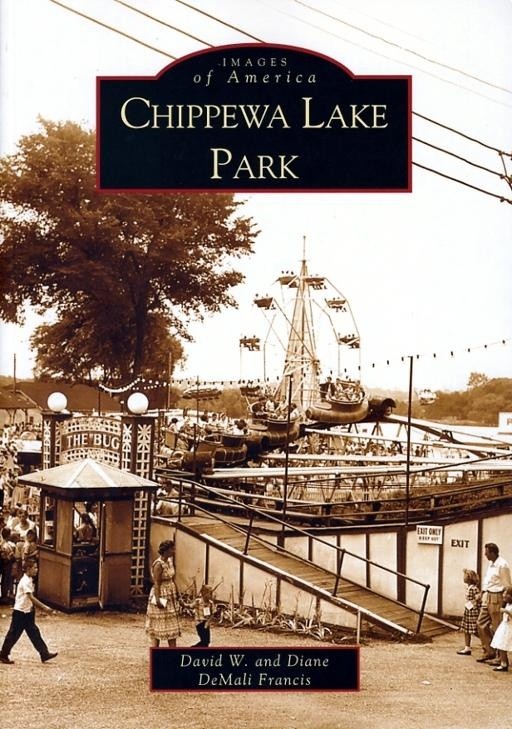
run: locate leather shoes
[42,654,57,661]
[457,649,471,654]
[478,652,508,671]
[1,657,13,663]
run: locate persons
[454,566,481,655]
[189,381,218,389]
[142,537,186,650]
[0,501,101,604]
[163,479,187,516]
[291,438,429,465]
[474,541,512,663]
[322,377,362,401]
[241,379,261,388]
[81,412,118,428]
[1,555,61,665]
[257,394,299,420]
[199,413,249,436]
[178,574,227,649]
[489,584,511,674]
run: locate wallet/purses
[150,596,167,608]
